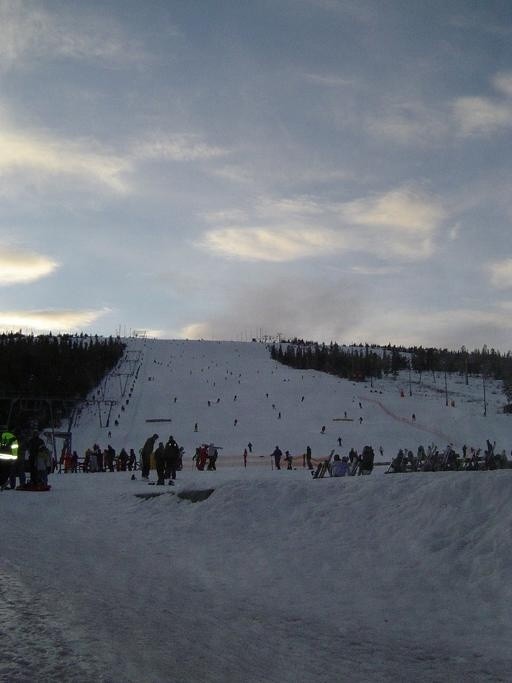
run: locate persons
[0,423,53,486]
[63,432,224,485]
[268,437,382,477]
[248,441,253,452]
